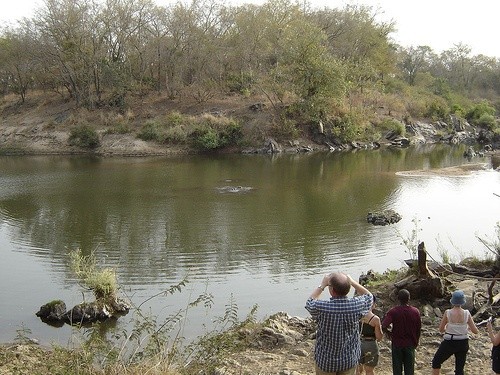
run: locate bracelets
[318,285,324,291]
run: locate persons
[431,290,479,375]
[486,319,500,375]
[356,295,384,375]
[305,271,373,375]
[381,288,421,375]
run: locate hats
[450,290,465,305]
